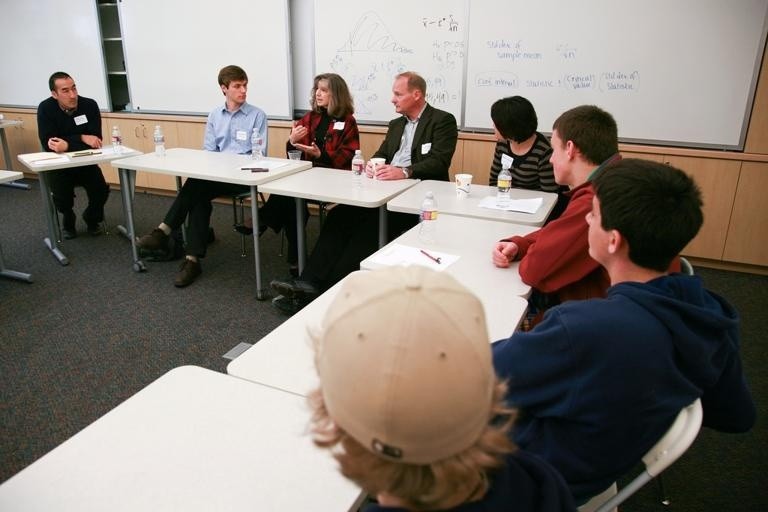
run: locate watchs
[401,167,410,179]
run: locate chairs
[230,194,267,257]
[678,255,695,276]
[577,397,704,511]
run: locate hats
[316,262,497,465]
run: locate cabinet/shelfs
[0,108,208,197]
[96,0,132,112]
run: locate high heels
[232,223,267,238]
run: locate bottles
[351,150,364,193]
[153,126,166,157]
[421,191,438,246]
[251,127,263,161]
[111,126,123,154]
[497,164,512,208]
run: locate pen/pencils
[242,168,262,170]
[73,153,92,158]
[251,169,269,172]
[420,250,441,264]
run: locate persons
[134,65,268,288]
[270,71,458,314]
[492,104,682,333]
[36,72,110,239]
[233,73,360,275]
[488,158,756,506]
[489,96,561,195]
[308,264,580,510]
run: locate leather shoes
[270,281,319,299]
[273,296,304,315]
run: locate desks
[0,170,33,283]
[0,365,368,512]
[17,144,145,266]
[111,147,312,300]
[225,268,529,399]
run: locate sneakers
[62,210,77,240]
[89,222,105,235]
[174,259,202,287]
[135,228,170,249]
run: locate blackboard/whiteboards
[310,2,463,133]
[115,0,296,120]
[461,0,768,152]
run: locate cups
[288,150,302,164]
[370,158,386,180]
[455,174,472,200]
